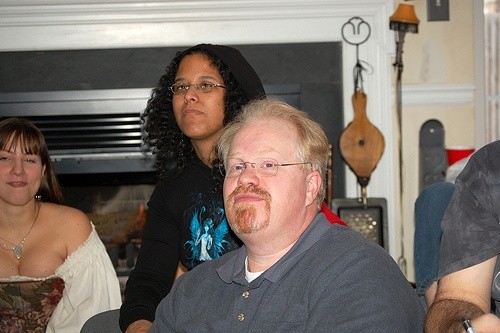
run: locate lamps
[389,2,419,69]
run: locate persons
[78,44,269,333]
[146,100,500,333]
[0,118,121,333]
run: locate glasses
[214,157,312,178]
[167,81,226,94]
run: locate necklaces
[0,200,39,261]
